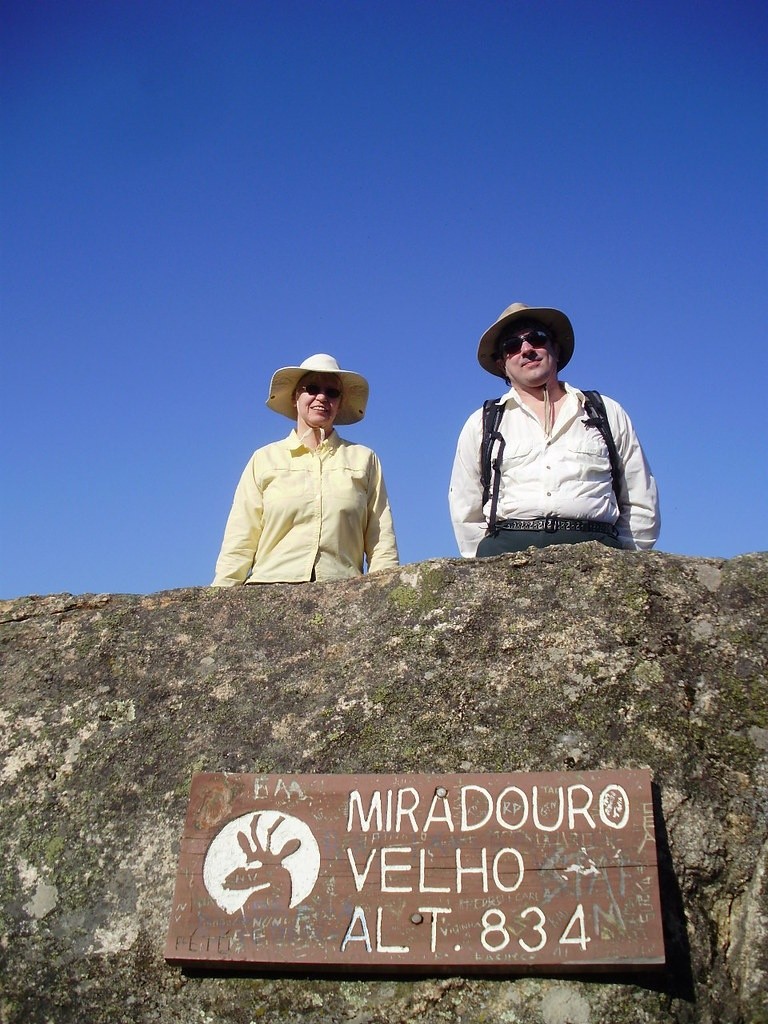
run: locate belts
[495,518,613,534]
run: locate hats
[265,354,369,425]
[477,303,574,381]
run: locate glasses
[297,384,343,399]
[501,331,553,360]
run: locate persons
[211,354,399,588]
[448,303,661,556]
